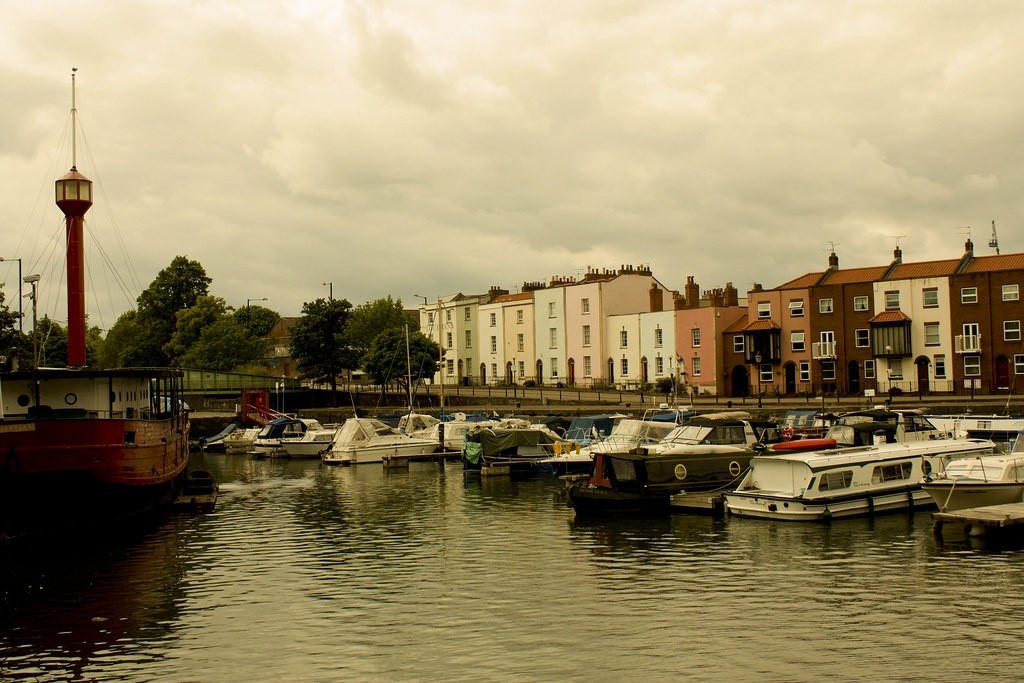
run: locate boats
[172,468,219,514]
[725,437,997,523]
[280,423,338,459]
[918,450,1024,512]
[321,417,442,465]
[247,417,324,458]
[462,403,1024,514]
[188,424,239,454]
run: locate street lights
[247,298,268,329]
[414,295,427,305]
[755,351,762,408]
[668,354,674,387]
[886,346,892,401]
[0,258,22,331]
[323,283,332,300]
[512,355,516,397]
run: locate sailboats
[399,300,531,451]
[0,75,192,545]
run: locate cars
[222,428,264,455]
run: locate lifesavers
[553,440,562,455]
[565,440,580,455]
[783,427,794,440]
